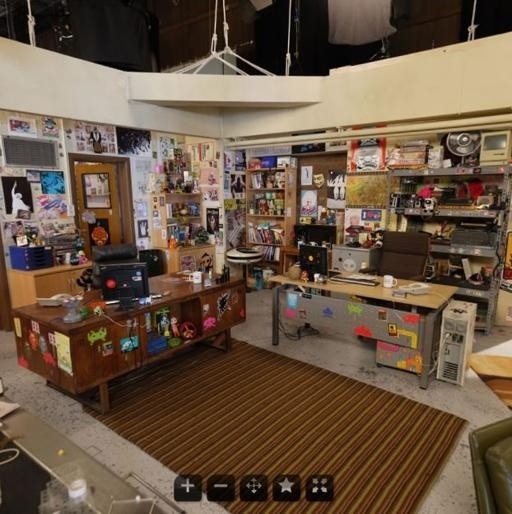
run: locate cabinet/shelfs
[5,260,92,311]
[382,163,512,336]
[149,191,216,273]
[245,167,298,275]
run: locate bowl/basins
[104,300,120,312]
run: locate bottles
[320,208,327,224]
[170,234,176,249]
[67,478,97,514]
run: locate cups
[190,271,203,284]
[383,275,397,288]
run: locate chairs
[377,229,430,281]
[89,242,139,289]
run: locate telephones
[397,282,430,295]
[36,293,73,306]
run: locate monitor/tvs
[479,130,512,165]
[299,244,329,280]
[99,263,150,309]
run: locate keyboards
[104,293,162,306]
[331,277,380,286]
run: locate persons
[231,177,246,198]
[88,127,104,153]
[11,180,30,218]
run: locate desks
[267,270,458,386]
[10,268,243,412]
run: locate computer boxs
[435,298,478,388]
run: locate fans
[446,132,480,167]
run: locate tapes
[67,479,87,498]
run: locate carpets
[81,338,467,512]
